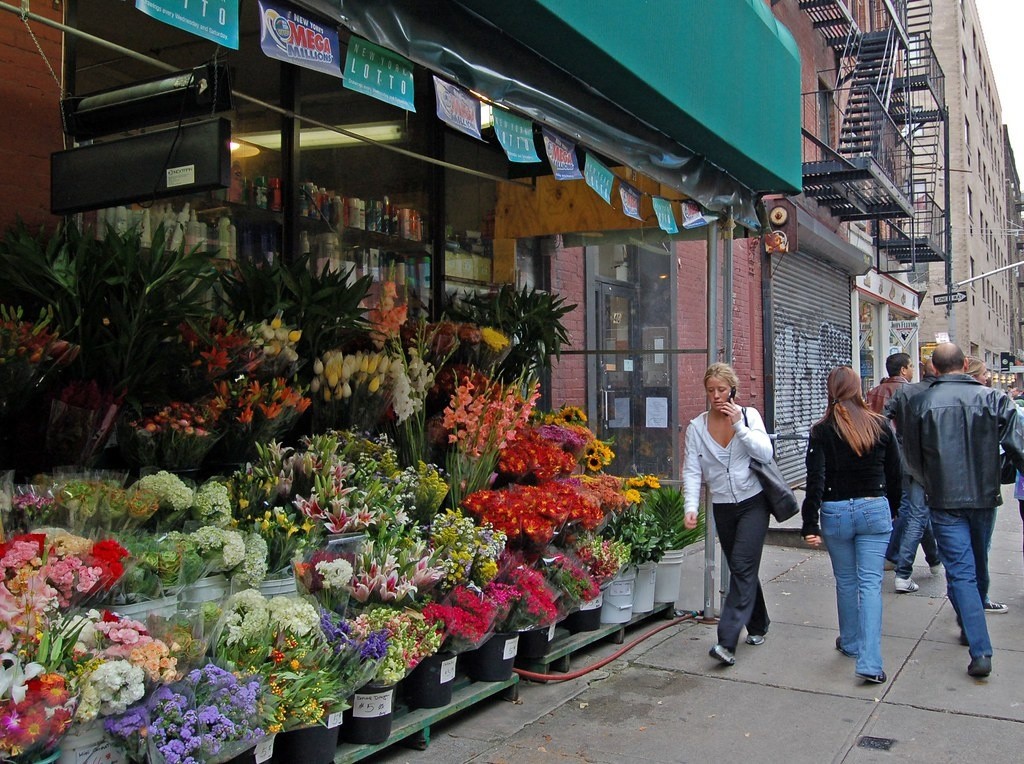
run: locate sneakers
[854,670,887,683]
[836,636,858,658]
[894,576,919,592]
[709,644,736,666]
[983,600,1008,614]
[746,633,766,646]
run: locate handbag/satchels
[742,405,799,523]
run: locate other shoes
[884,558,898,571]
[931,562,942,574]
[968,654,992,676]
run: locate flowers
[0,214,713,764]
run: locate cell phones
[724,386,736,416]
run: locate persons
[867,344,1024,680]
[800,367,903,684]
[681,362,774,665]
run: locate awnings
[63,0,802,240]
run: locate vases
[655,550,683,602]
[599,573,635,622]
[515,624,556,667]
[558,588,604,632]
[338,684,396,746]
[459,630,518,683]
[15,745,62,764]
[258,578,297,600]
[396,649,459,709]
[630,563,656,611]
[56,718,130,764]
[268,723,338,764]
[90,593,178,627]
[178,573,228,616]
[168,467,201,483]
[201,460,242,482]
